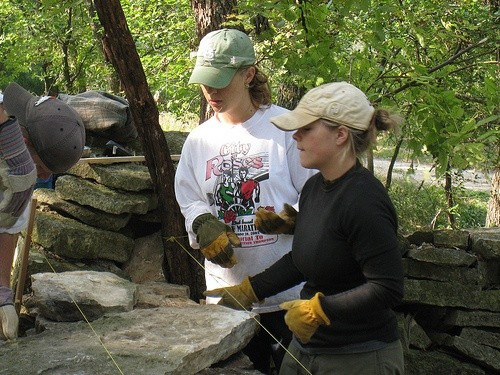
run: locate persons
[173,27,321,374]
[0,82,85,344]
[202,82,409,375]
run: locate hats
[270,80,375,131]
[186,28,254,89]
[2,83,86,173]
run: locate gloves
[279,292,330,344]
[202,275,260,311]
[192,212,241,268]
[254,203,299,236]
[0,287,19,341]
